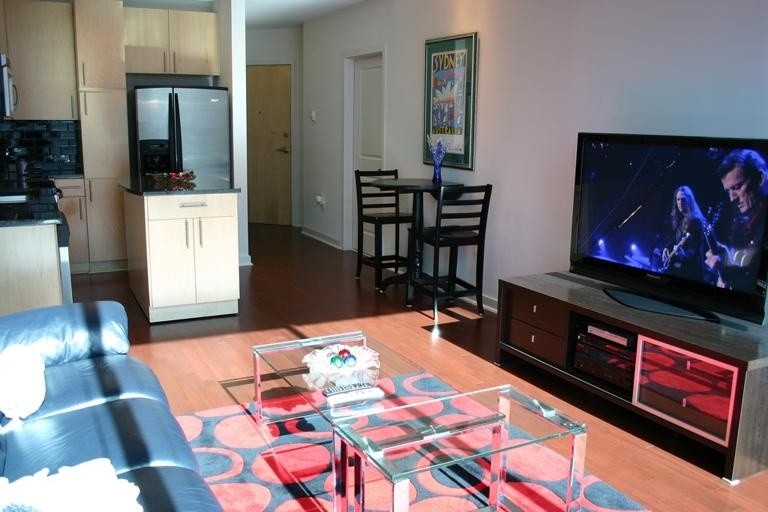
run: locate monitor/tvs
[570,132,768,325]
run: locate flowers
[423,131,456,168]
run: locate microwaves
[2,54,19,117]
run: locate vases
[433,162,443,185]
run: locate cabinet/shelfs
[55,178,93,276]
[496,272,768,484]
[122,190,245,324]
[74,0,126,90]
[75,87,132,177]
[3,2,78,122]
[85,176,130,275]
[122,5,221,78]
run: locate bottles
[16,155,29,175]
[17,175,30,189]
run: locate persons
[705,150,768,294]
[659,186,709,283]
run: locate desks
[374,178,469,312]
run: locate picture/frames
[419,30,479,170]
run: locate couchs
[0,297,230,509]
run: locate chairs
[403,180,492,321]
[352,165,419,298]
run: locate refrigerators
[135,85,231,188]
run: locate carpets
[171,366,646,512]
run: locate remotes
[327,387,384,405]
[322,382,374,396]
